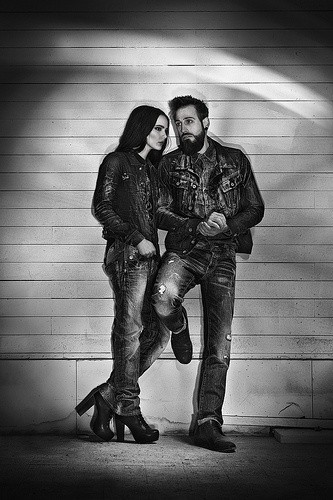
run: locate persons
[75,104,212,444]
[151,95,266,451]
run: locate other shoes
[194,415,237,451]
[171,307,193,364]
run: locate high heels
[74,388,115,442]
[115,413,159,443]
